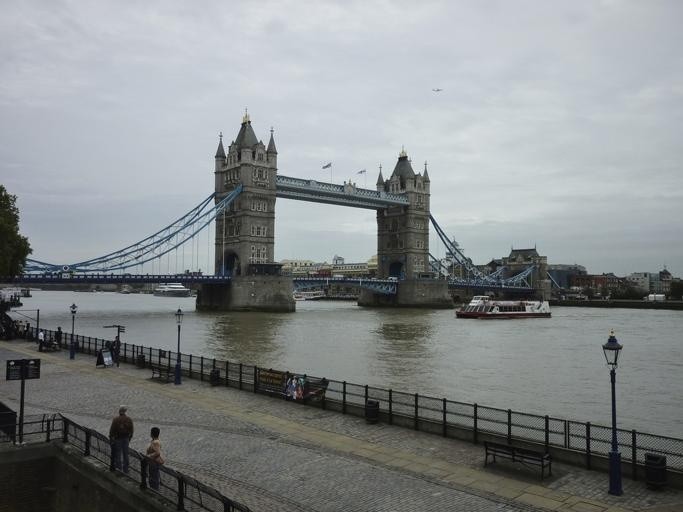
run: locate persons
[0,319,31,341]
[54,326,63,348]
[111,335,122,364]
[108,405,135,475]
[37,328,45,353]
[140,426,165,493]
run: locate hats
[120,406,127,415]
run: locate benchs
[482,435,553,480]
[151,363,175,382]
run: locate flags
[322,162,332,169]
[357,170,366,174]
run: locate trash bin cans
[210,369,220,386]
[75,342,79,351]
[136,355,145,368]
[365,399,380,425]
[645,452,667,491]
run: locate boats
[455,295,552,319]
[152,281,192,297]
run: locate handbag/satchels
[151,457,164,466]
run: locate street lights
[69,303,78,360]
[172,304,185,386]
[601,328,625,496]
